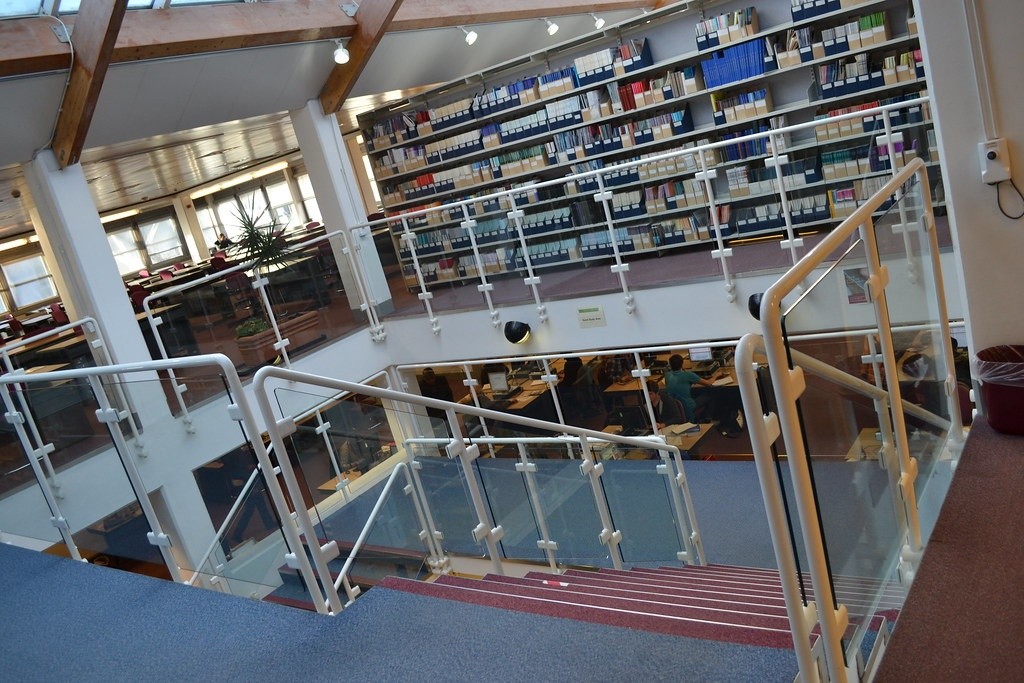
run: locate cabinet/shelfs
[355,0,924,296]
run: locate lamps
[333,40,350,64]
[591,14,605,29]
[458,26,478,46]
[544,18,560,36]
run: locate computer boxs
[492,385,520,400]
[691,361,720,378]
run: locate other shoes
[266,520,277,530]
[232,536,243,543]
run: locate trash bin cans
[976,345,1024,437]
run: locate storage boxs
[373,0,941,290]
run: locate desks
[894,327,939,397]
[318,447,399,495]
[0,217,324,453]
[203,434,270,502]
[87,506,145,560]
[470,344,758,460]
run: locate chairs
[270,232,283,236]
[272,238,289,251]
[227,272,265,320]
[673,397,702,424]
[159,271,174,280]
[50,303,62,312]
[210,257,229,272]
[52,310,69,325]
[213,250,228,259]
[130,285,144,293]
[318,242,343,291]
[306,222,320,230]
[567,360,597,417]
[132,290,154,313]
[5,314,14,320]
[8,320,26,337]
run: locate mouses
[512,400,518,404]
[345,471,350,474]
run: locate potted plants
[226,187,322,367]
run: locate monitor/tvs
[687,348,714,367]
[487,372,509,393]
[356,432,381,462]
[535,359,551,370]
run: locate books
[364,1,942,287]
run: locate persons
[218,233,232,250]
[664,354,722,413]
[861,334,885,391]
[481,363,509,387]
[226,447,279,542]
[643,380,677,428]
[952,336,971,386]
[418,368,454,426]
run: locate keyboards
[354,461,367,472]
[494,400,511,408]
